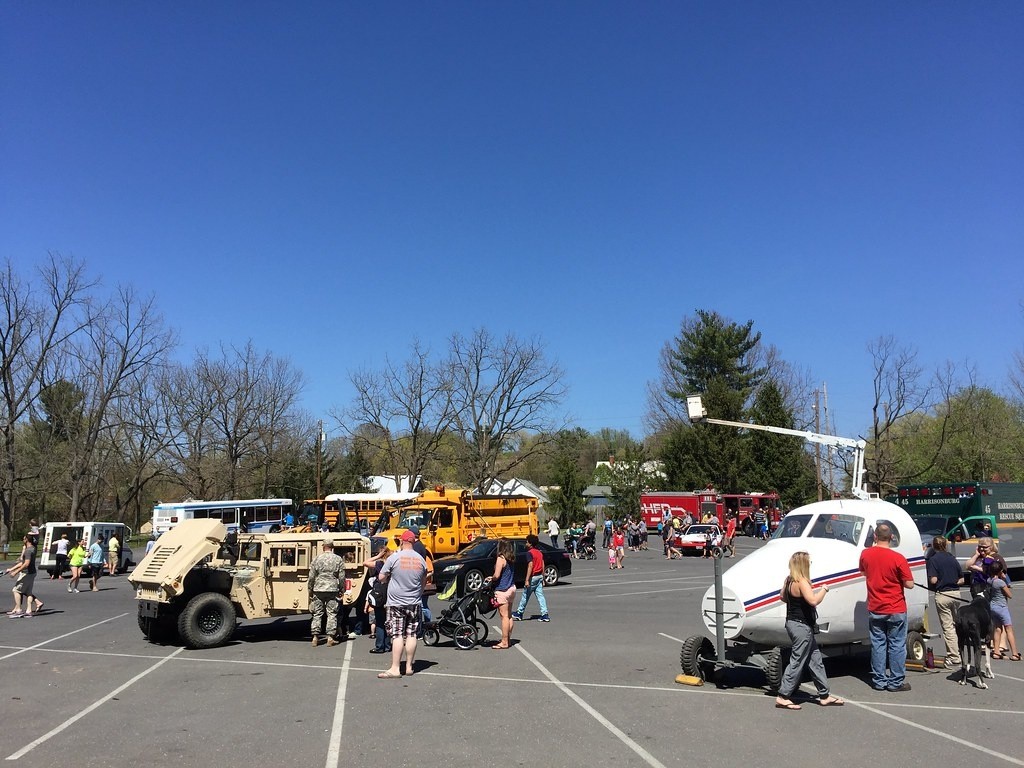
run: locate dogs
[953,590,992,689]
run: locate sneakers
[891,683,911,691]
[512,611,523,620]
[538,614,549,622]
[66,586,79,593]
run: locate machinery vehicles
[128,517,372,650]
[369,489,541,562]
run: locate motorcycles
[563,524,586,552]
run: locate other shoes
[109,573,116,576]
[35,603,44,613]
[51,576,55,579]
[701,556,713,558]
[7,609,24,615]
[346,630,392,654]
[10,611,32,618]
[311,637,318,646]
[89,580,98,591]
[58,576,63,580]
[327,637,339,647]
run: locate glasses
[978,545,992,549]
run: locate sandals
[989,646,1023,661]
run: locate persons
[859,525,914,692]
[67,539,88,593]
[511,534,550,622]
[545,512,649,570]
[775,552,845,710]
[50,534,70,579]
[927,536,964,665]
[283,512,434,679]
[658,507,798,559]
[87,535,107,592]
[145,535,156,556]
[108,533,120,576]
[6,519,44,619]
[485,538,516,649]
[965,522,1022,661]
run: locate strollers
[577,536,597,560]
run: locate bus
[152,498,293,533]
[323,493,423,530]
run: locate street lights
[811,388,823,502]
[317,419,327,499]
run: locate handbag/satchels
[478,594,498,619]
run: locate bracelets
[823,587,829,592]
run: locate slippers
[820,696,844,706]
[776,703,802,710]
[492,639,512,649]
[377,667,414,678]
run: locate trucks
[886,481,1024,586]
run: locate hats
[321,539,333,546]
[397,531,415,541]
[409,526,420,535]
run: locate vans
[37,522,136,578]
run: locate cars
[665,524,725,555]
[432,537,572,593]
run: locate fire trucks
[640,489,725,530]
[719,492,784,536]
